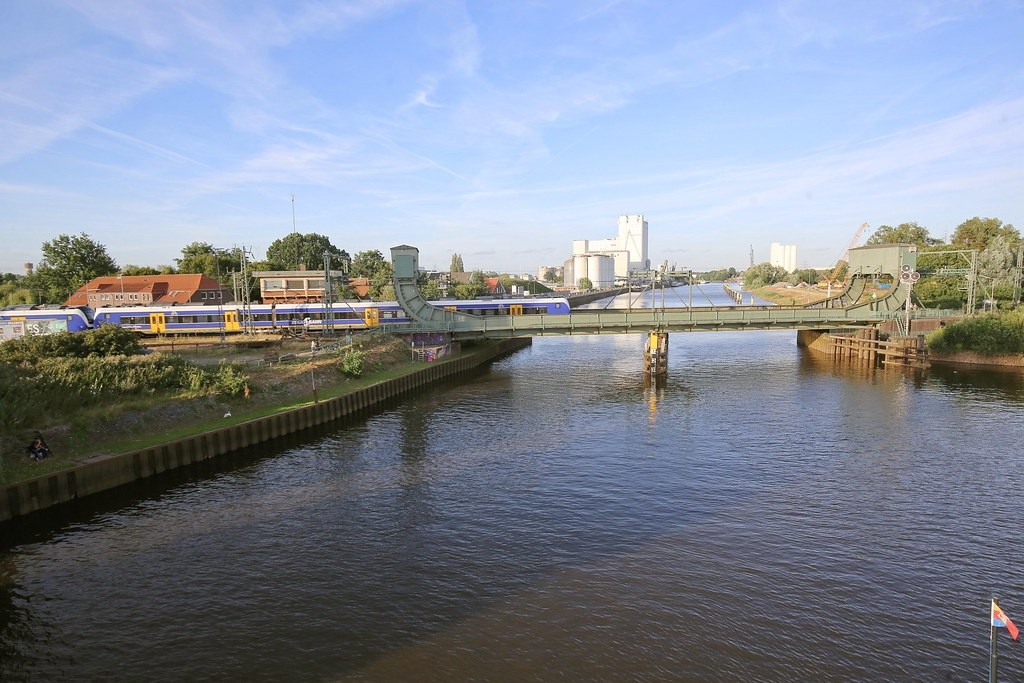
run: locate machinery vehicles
[817,222,870,288]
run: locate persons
[311,336,319,351]
[345,325,354,344]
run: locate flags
[991,599,1019,640]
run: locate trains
[0,298,571,339]
